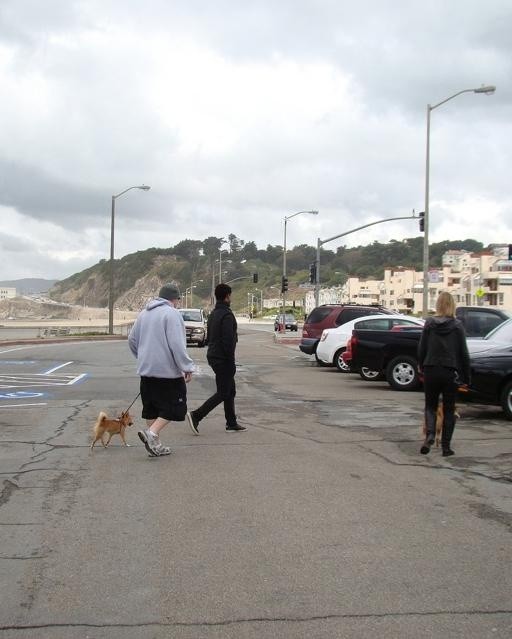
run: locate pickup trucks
[351,307,509,391]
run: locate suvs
[299,303,400,367]
[177,309,209,347]
[274,313,298,331]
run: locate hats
[159,283,179,300]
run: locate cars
[343,325,426,383]
[467,345,512,419]
[460,317,512,353]
[315,316,427,374]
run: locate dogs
[90,411,133,450]
[422,402,460,447]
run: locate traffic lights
[254,274,257,283]
[509,244,512,260]
[282,276,288,293]
[309,264,317,284]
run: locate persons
[184,282,248,435]
[415,290,472,456]
[127,282,197,457]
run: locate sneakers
[138,411,247,456]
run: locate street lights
[213,271,228,305]
[180,280,203,308]
[423,84,496,315]
[212,260,232,306]
[281,210,318,313]
[247,292,254,320]
[255,288,263,318]
[109,185,151,332]
[219,246,243,285]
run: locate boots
[421,410,437,454]
[442,418,456,456]
